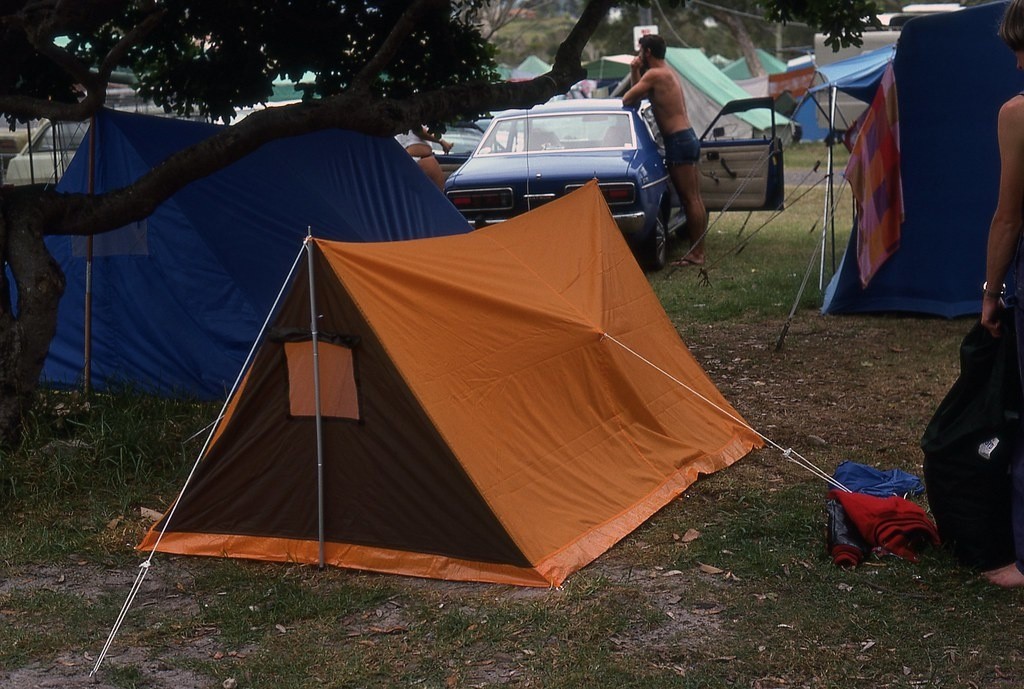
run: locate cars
[425,96,785,270]
[0,70,325,187]
[426,119,519,155]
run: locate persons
[981,0,1024,588]
[394,124,453,194]
[621,34,708,267]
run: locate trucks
[814,29,900,156]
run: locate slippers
[669,257,705,266]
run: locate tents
[37,0,1024,403]
[136,178,764,590]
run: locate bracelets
[981,281,1006,298]
